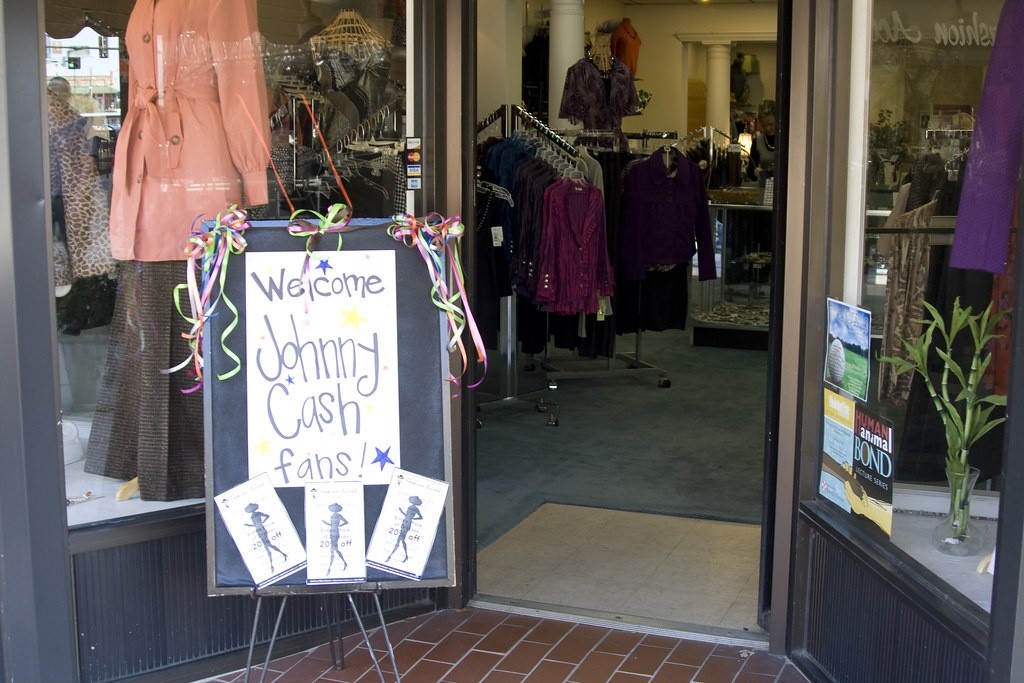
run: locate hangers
[268,75,397,208]
[475,100,748,192]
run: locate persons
[105,0,270,501]
[747,99,776,188]
[610,17,642,77]
[46,75,119,300]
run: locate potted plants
[874,294,1016,556]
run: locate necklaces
[764,135,775,149]
